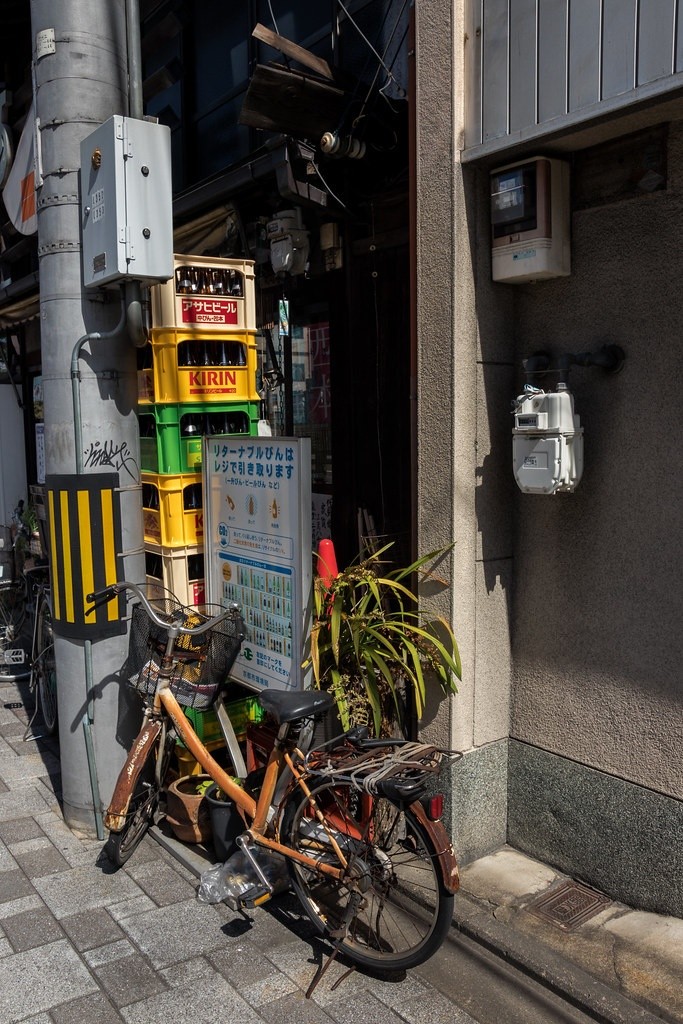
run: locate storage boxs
[124,252,262,618]
[165,675,264,750]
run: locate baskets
[122,599,245,711]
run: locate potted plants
[164,771,250,860]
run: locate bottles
[136,268,245,435]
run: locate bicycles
[84,582,461,998]
[0,521,60,742]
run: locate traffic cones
[317,539,339,619]
